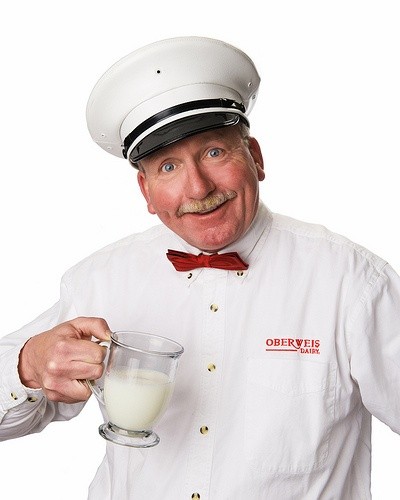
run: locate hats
[86,36,261,167]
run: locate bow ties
[165,249,248,271]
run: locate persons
[0,36,400,500]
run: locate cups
[86,330,184,448]
